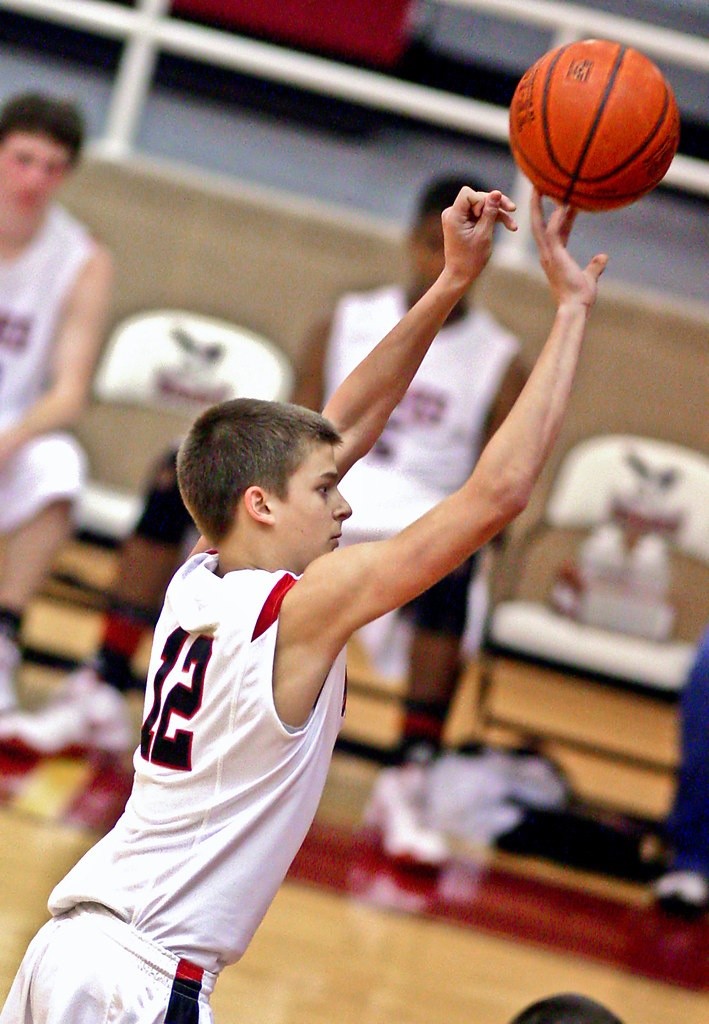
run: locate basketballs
[509,39,680,215]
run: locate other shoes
[653,869,709,921]
[0,637,20,710]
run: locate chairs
[19,309,709,862]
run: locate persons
[0,93,708,923]
[0,185,608,1024]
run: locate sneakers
[0,668,134,755]
[362,765,451,864]
